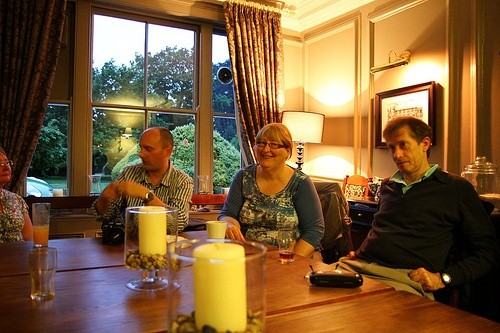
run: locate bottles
[461,156,500,214]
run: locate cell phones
[309,270,363,289]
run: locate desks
[346,199,379,251]
[0,231,500,333]
[24,209,223,240]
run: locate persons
[330,118,500,302]
[92,126,195,234]
[0,148,34,243]
[217,123,325,263]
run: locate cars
[26,176,54,197]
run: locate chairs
[342,175,373,201]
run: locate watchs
[143,190,154,204]
[442,272,452,285]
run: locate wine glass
[123,206,179,292]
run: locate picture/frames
[375,80,436,149]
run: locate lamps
[280,110,325,170]
[369,50,410,73]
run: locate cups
[28,248,57,301]
[32,203,50,248]
[278,229,296,264]
[206,221,227,240]
[167,238,267,333]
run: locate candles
[138,206,167,256]
[192,243,247,333]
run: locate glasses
[0,160,14,167]
[256,141,286,149]
[304,263,339,286]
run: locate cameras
[101,221,125,246]
